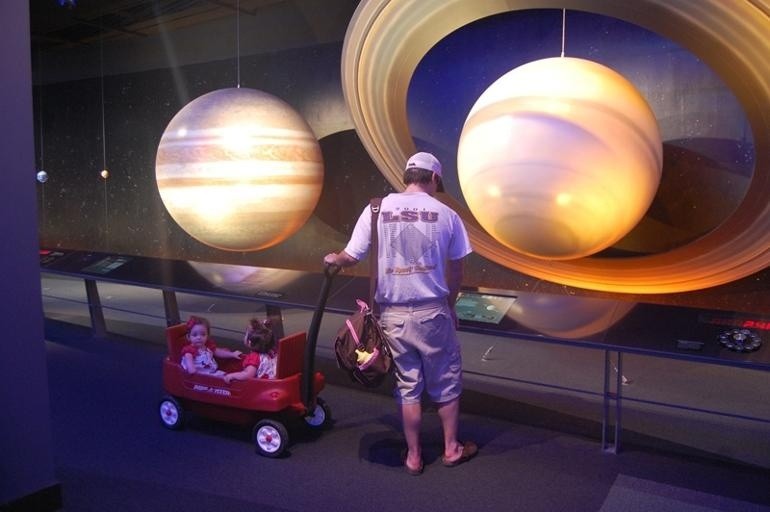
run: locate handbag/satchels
[333,299,395,389]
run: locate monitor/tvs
[452,290,517,325]
[40,250,63,266]
[81,254,131,275]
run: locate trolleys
[156,260,341,459]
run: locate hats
[405,151,447,194]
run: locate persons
[322,151,478,474]
[224,317,282,385]
[177,314,242,377]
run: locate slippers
[403,447,425,475]
[441,439,479,467]
